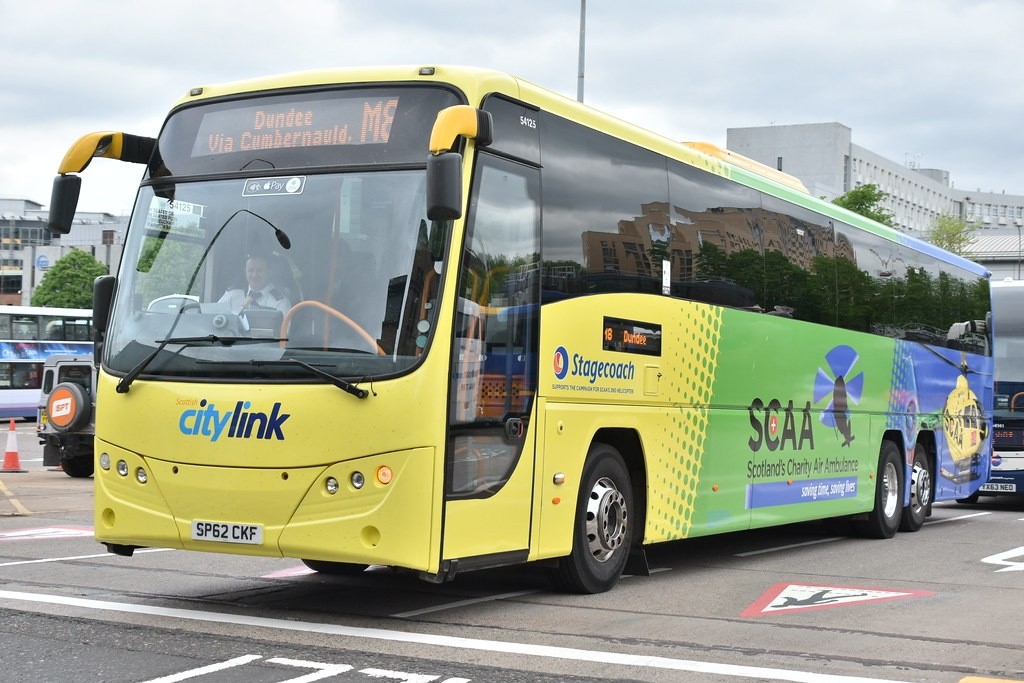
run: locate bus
[47,62,997,596]
[0,306,99,420]
[955,278,1024,504]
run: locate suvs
[37,355,101,478]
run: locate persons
[217,255,291,333]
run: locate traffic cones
[0,419,29,473]
